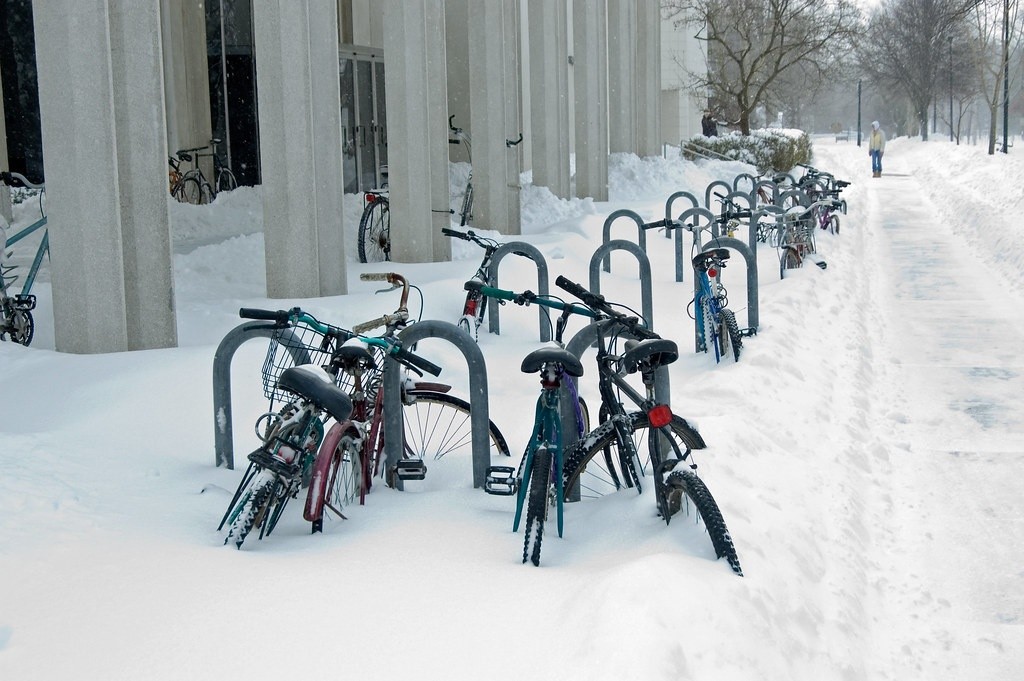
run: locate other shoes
[873,170,881,177]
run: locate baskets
[766,218,812,247]
[261,321,385,411]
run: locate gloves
[879,152,884,158]
[869,151,871,156]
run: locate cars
[835,133,849,142]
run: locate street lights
[947,36,953,142]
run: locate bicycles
[713,162,853,282]
[357,138,462,264]
[0,172,51,347]
[167,152,202,206]
[996,138,1014,153]
[218,305,442,553]
[181,139,237,205]
[300,270,512,536]
[556,273,744,580]
[449,115,524,226]
[638,210,755,362]
[508,286,649,569]
[440,226,535,343]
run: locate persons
[701,110,717,138]
[869,121,886,178]
